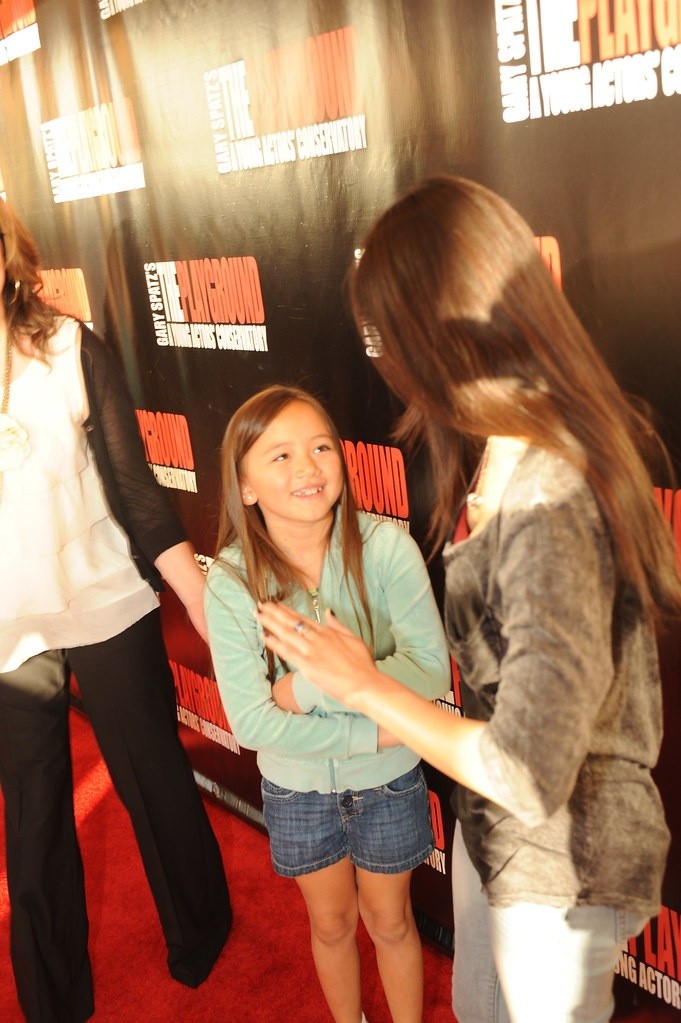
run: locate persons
[204,386,452,1023]
[0,200,209,1023]
[255,175,681,1023]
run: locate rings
[295,621,310,632]
